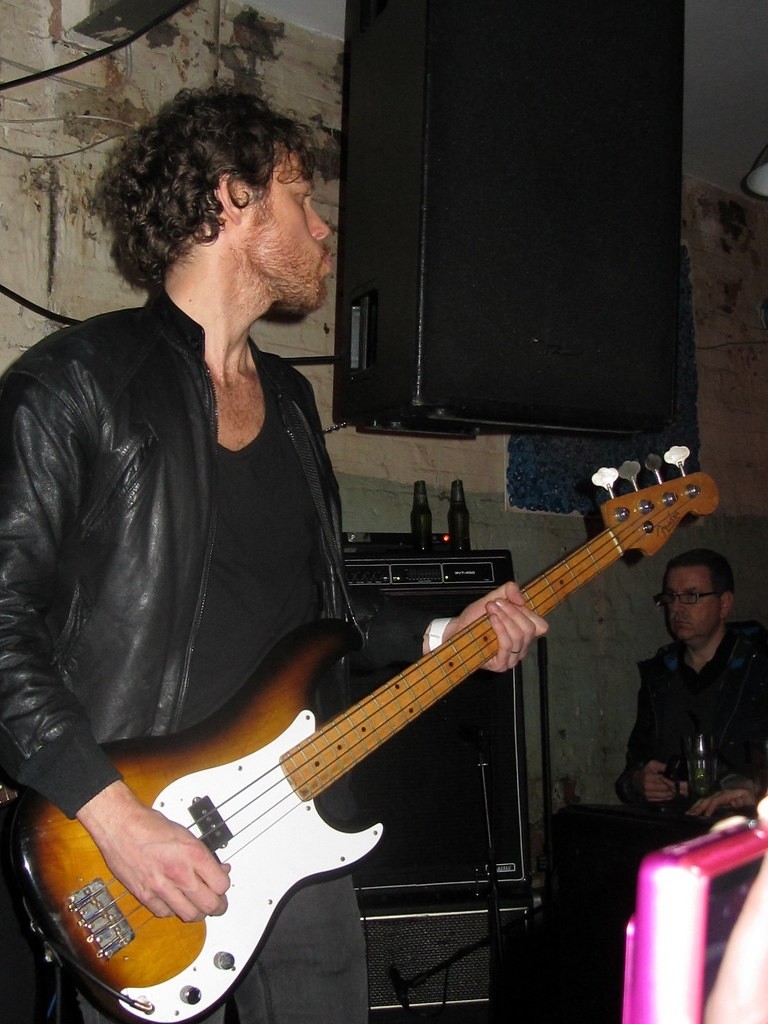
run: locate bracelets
[428,617,450,653]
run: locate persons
[2,96,552,1023]
[614,544,767,817]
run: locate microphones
[388,967,409,1008]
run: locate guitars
[7,444,722,1024]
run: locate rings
[511,650,521,653]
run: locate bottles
[448,479,471,556]
[410,480,432,558]
[687,735,715,801]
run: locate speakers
[358,898,530,1010]
[332,0,685,438]
[312,585,532,892]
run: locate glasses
[653,592,717,606]
